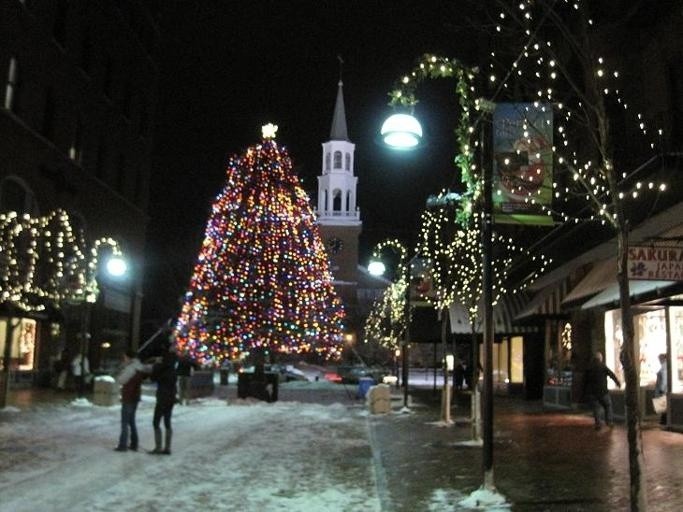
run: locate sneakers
[114,446,126,451]
[128,446,136,450]
[148,449,170,455]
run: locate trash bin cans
[94,376,116,404]
[359,378,374,398]
[366,383,391,414]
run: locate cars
[238,363,386,387]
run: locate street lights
[77,235,127,399]
[368,240,410,414]
[380,50,508,509]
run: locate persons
[453,363,463,390]
[581,351,621,430]
[68,350,90,396]
[112,351,147,451]
[146,348,177,453]
[175,353,193,405]
[463,364,473,389]
[654,353,668,424]
